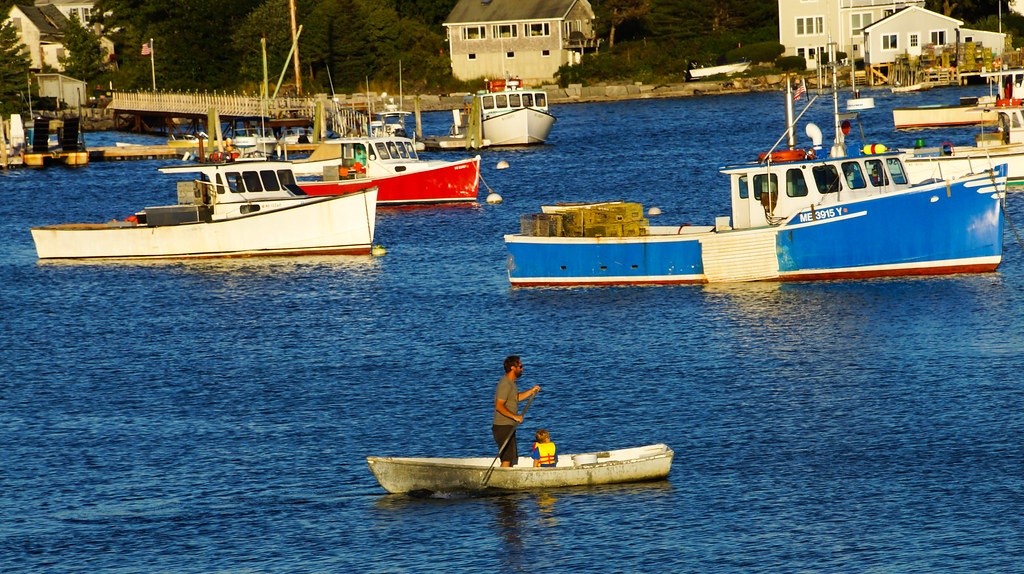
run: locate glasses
[515,365,522,369]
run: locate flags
[794,81,805,101]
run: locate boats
[684,60,752,82]
[365,442,674,496]
[477,78,557,146]
[156,60,482,205]
[504,60,1007,292]
[29,89,380,261]
[857,68,1024,186]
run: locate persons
[327,122,340,138]
[532,431,557,466]
[834,121,851,143]
[298,131,309,143]
[493,356,540,467]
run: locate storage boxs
[519,200,650,238]
